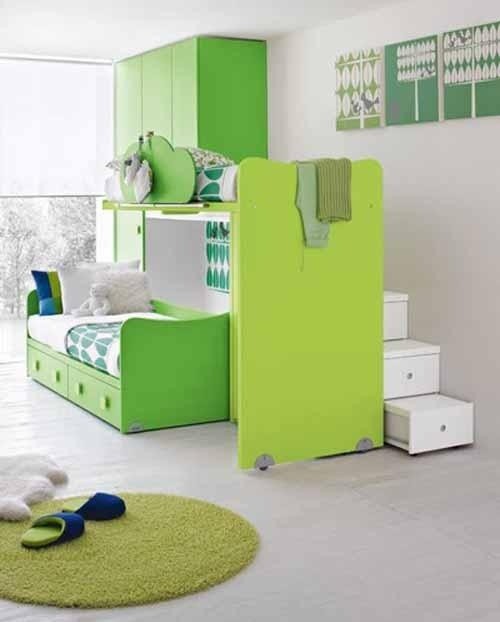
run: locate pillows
[31,268,153,316]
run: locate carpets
[0,490,260,610]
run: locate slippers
[63,491,126,520]
[20,511,85,547]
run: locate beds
[24,262,231,434]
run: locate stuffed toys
[70,281,113,317]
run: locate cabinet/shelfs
[110,35,477,473]
